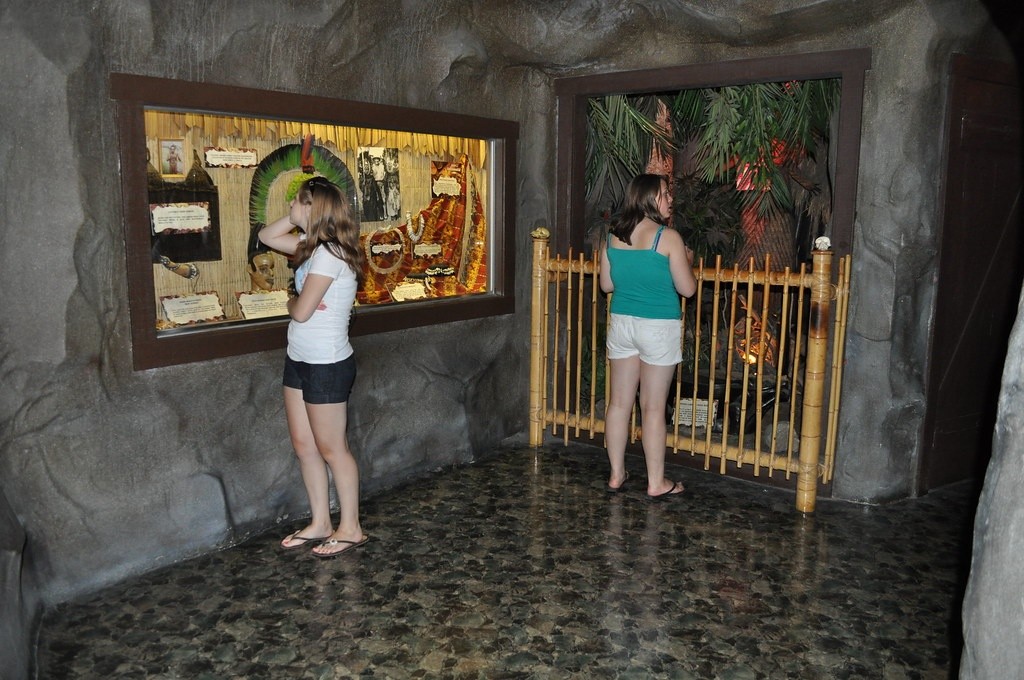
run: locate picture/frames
[158,136,186,179]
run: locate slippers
[280,528,334,550]
[606,469,630,493]
[644,475,688,501]
[311,534,368,557]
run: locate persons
[257,176,369,558]
[246,251,275,293]
[359,152,401,221]
[166,145,179,173]
[599,173,697,498]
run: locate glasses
[309,176,328,199]
